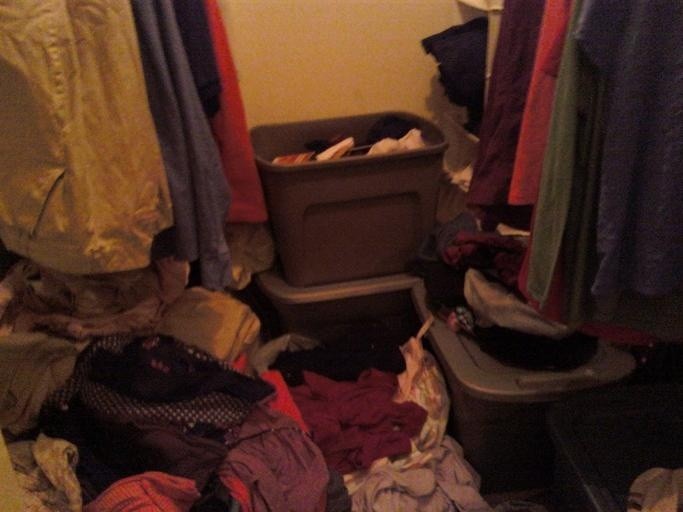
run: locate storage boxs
[249,108,451,282]
[410,288,644,491]
[252,261,409,359]
